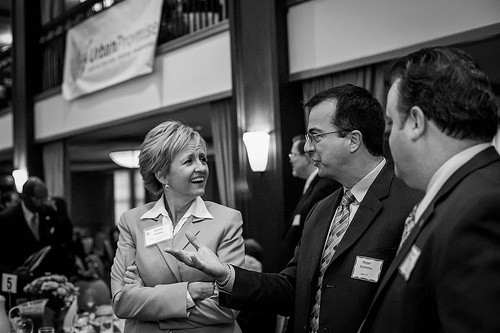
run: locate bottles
[0,295,11,333]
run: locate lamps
[243,131,270,173]
[109,150,140,168]
[12,170,28,194]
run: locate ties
[309,190,356,333]
[395,204,418,255]
[30,215,39,240]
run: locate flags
[61,0,164,101]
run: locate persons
[162,84,426,333]
[0,176,89,281]
[287,135,341,245]
[357,48,500,333]
[111,121,245,333]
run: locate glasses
[288,153,303,158]
[305,129,365,142]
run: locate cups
[14,319,34,333]
[38,327,55,333]
[99,317,114,333]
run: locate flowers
[24,275,80,305]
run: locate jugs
[7,298,49,333]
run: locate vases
[41,306,65,330]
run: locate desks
[10,298,126,333]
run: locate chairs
[74,271,110,305]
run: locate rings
[132,280,134,284]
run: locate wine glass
[86,289,96,320]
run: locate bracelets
[213,283,218,295]
[215,265,231,285]
